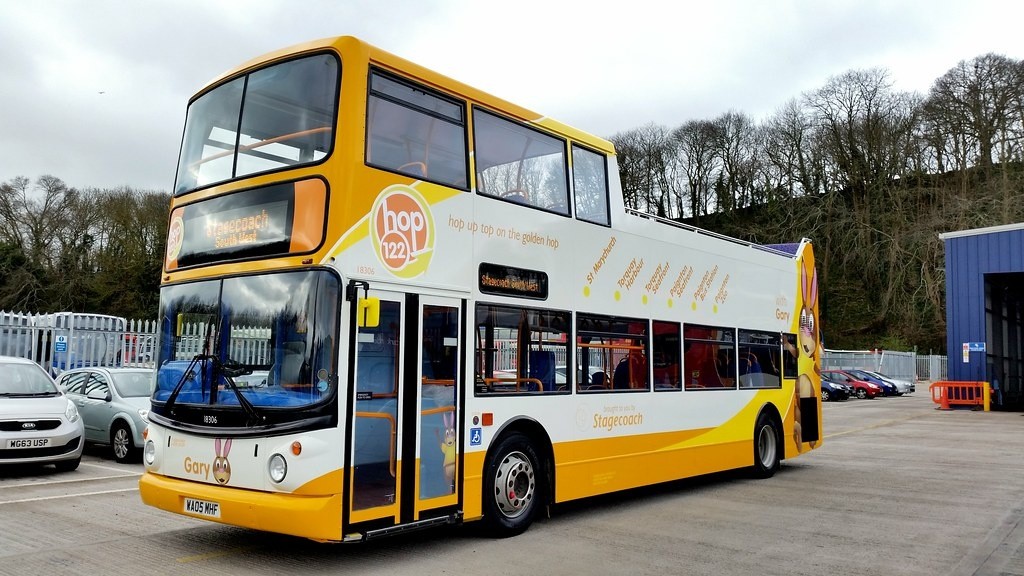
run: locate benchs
[477,353,765,394]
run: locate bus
[138,36,821,543]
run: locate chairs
[396,161,606,223]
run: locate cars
[821,369,883,399]
[0,356,85,472]
[821,374,850,401]
[853,370,899,397]
[866,371,916,395]
[56,366,155,463]
